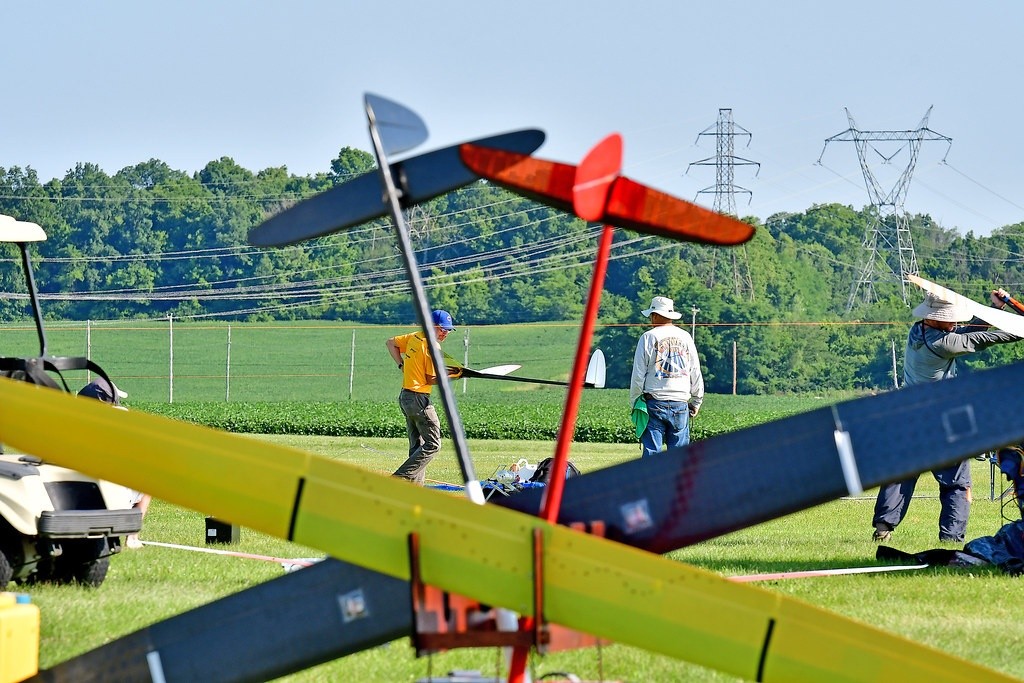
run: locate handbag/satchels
[529,458,581,486]
[509,459,538,482]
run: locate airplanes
[438,348,607,389]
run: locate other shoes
[872,530,891,542]
[126,539,143,548]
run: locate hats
[911,292,974,322]
[77,376,128,406]
[432,310,456,332]
[641,296,682,319]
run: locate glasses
[438,327,452,333]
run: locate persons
[76,378,151,549]
[629,297,704,460]
[386,309,463,484]
[871,289,1024,545]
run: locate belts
[401,388,415,393]
[644,393,654,400]
